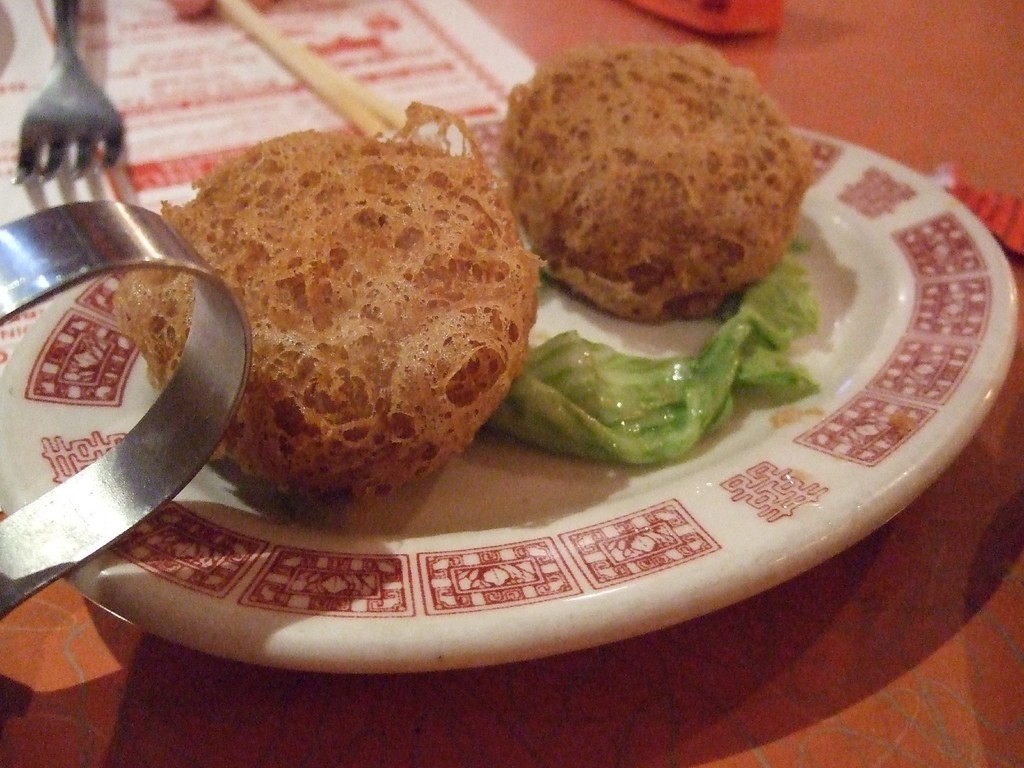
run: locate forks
[11,0,122,191]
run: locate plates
[64,122,1018,671]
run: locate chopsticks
[220,0,409,139]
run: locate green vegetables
[490,253,815,466]
